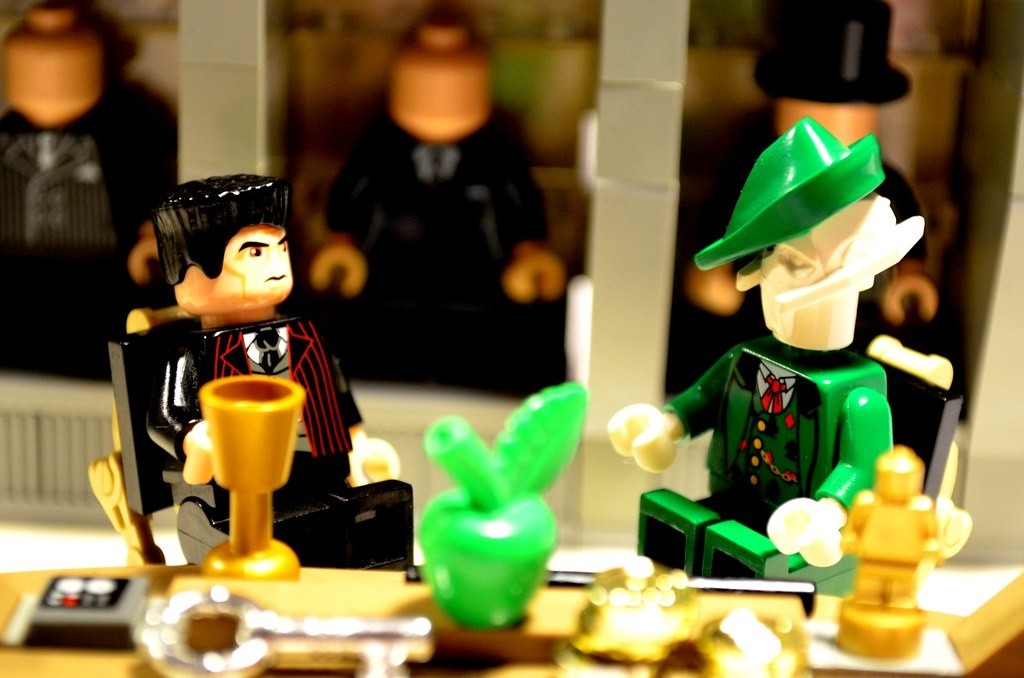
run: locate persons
[607,114,894,582]
[108,174,414,570]
[669,0,967,427]
[298,0,570,395]
[0,0,179,382]
